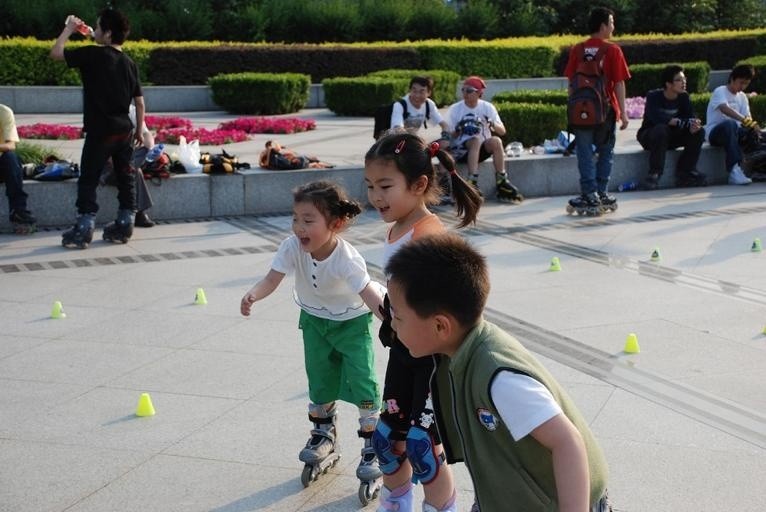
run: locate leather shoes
[135,212,155,227]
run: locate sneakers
[677,168,714,186]
[750,172,766,182]
[729,165,753,184]
[356,415,383,505]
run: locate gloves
[677,118,701,133]
[440,131,452,142]
[455,122,462,131]
[744,116,758,129]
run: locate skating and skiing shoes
[376,478,414,512]
[421,488,458,512]
[495,172,523,204]
[644,170,660,190]
[438,178,455,206]
[566,193,604,216]
[101,210,134,244]
[298,403,342,490]
[600,192,618,213]
[9,207,35,235]
[61,211,95,248]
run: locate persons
[364,133,483,511]
[384,236,616,512]
[391,76,451,150]
[49,8,146,253]
[705,63,765,186]
[0,104,39,235]
[636,65,706,190]
[239,179,385,507]
[446,76,524,204]
[99,105,163,229]
[563,7,631,215]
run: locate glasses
[460,86,477,94]
[673,77,687,81]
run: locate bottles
[619,182,636,191]
[146,144,164,162]
[529,145,543,154]
[65,15,96,37]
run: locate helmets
[461,114,481,135]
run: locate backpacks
[567,42,612,131]
[373,97,430,141]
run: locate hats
[464,77,486,97]
